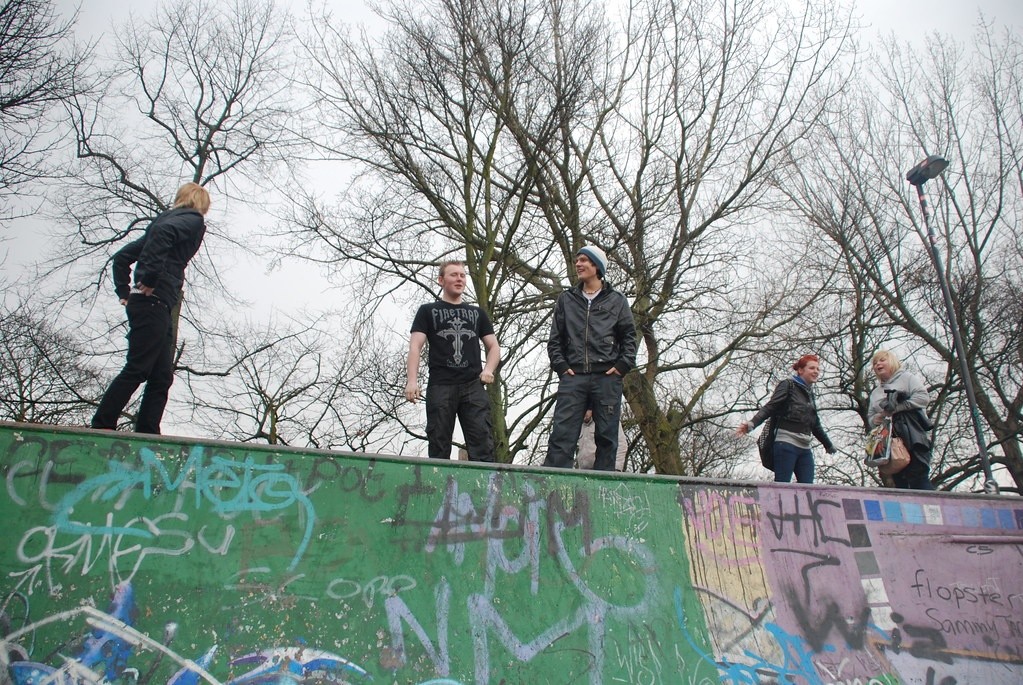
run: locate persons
[735,355,837,484]
[404,262,500,463]
[543,246,639,472]
[91,183,210,435]
[867,349,934,490]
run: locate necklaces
[582,285,603,294]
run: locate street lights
[906,154,1001,493]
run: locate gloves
[826,446,838,454]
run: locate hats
[577,246,608,277]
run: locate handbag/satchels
[758,414,776,473]
[864,416,893,467]
[879,428,910,475]
